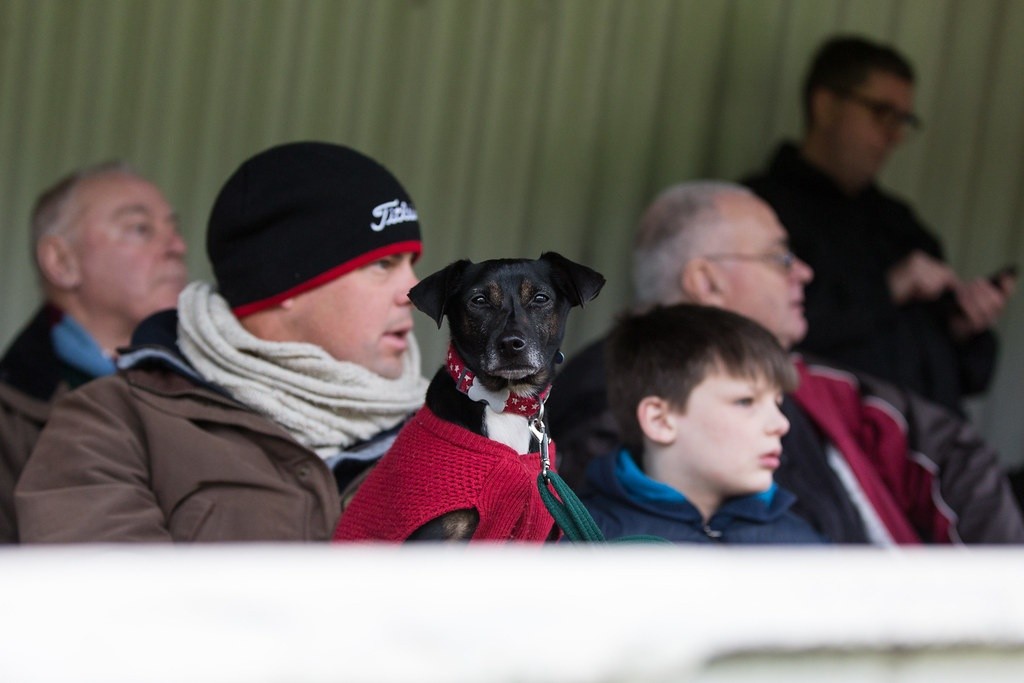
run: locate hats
[204,138,425,321]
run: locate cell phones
[951,264,1018,321]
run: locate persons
[0,161,190,545]
[739,34,1013,414]
[576,302,823,546]
[547,180,966,547]
[16,142,438,542]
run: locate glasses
[823,80,924,143]
[698,244,796,273]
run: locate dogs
[326,250,609,547]
